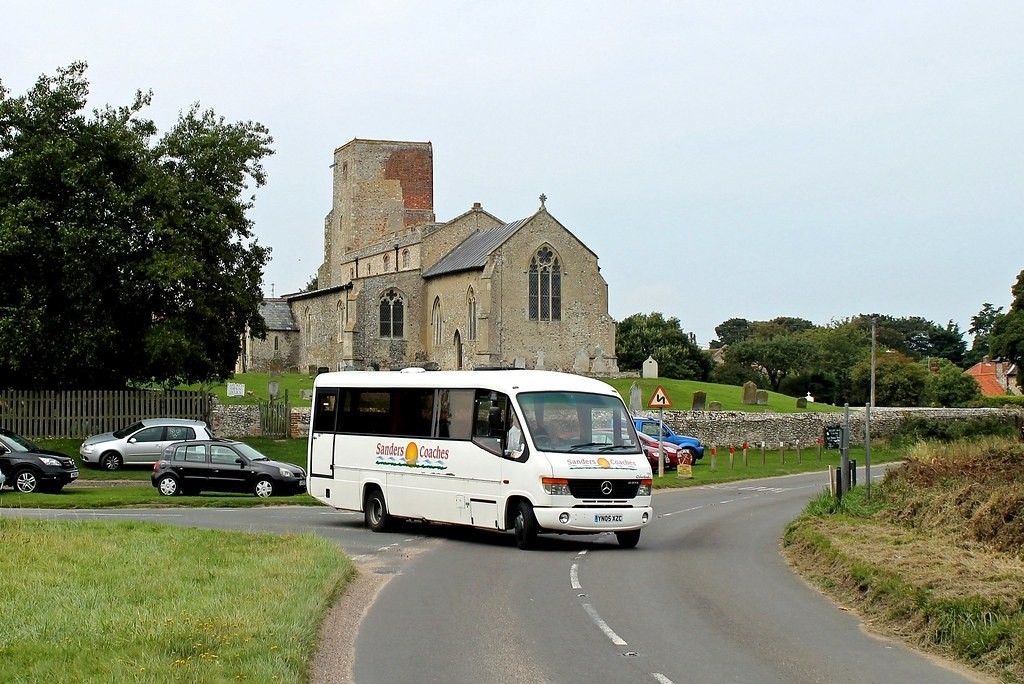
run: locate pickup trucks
[610,416,705,467]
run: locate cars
[559,431,670,475]
[151,437,306,498]
[79,418,215,473]
[595,427,690,468]
[0,428,80,494]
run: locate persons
[504,412,542,454]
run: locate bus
[306,366,653,550]
